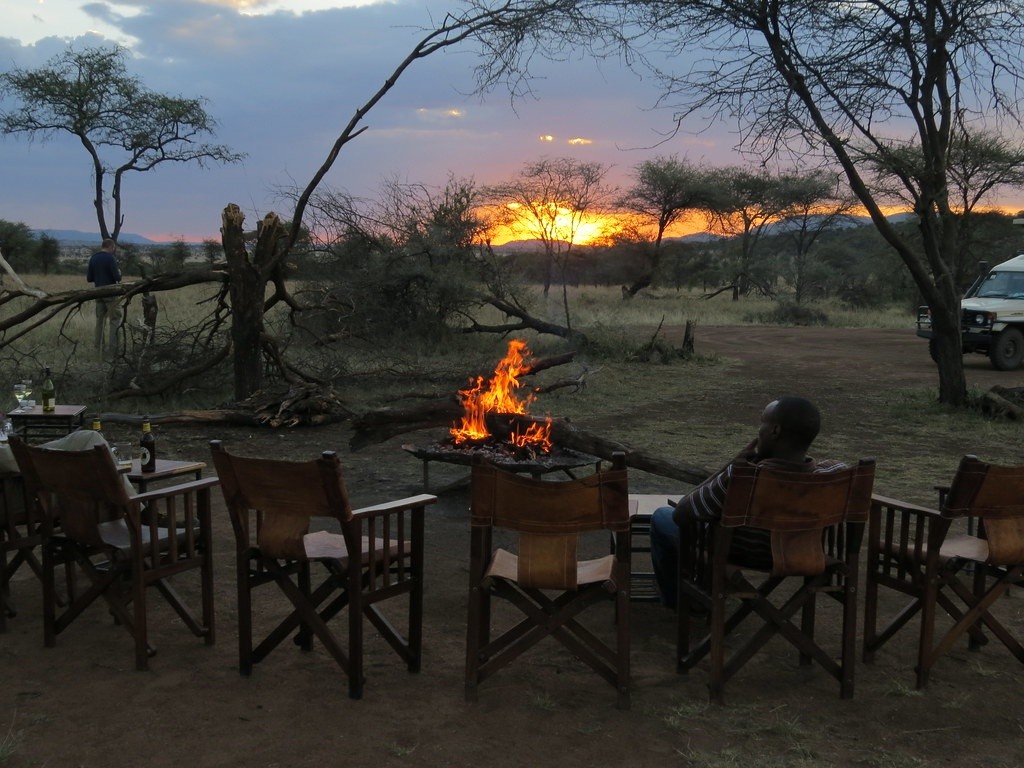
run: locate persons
[87,238,123,354]
[50,431,199,624]
[650,395,849,621]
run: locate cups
[111,442,133,468]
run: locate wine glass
[21,379,33,410]
[13,384,26,413]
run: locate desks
[617,493,694,630]
[8,405,88,445]
[118,458,206,521]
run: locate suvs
[915,253,1024,370]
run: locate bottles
[141,417,155,472]
[42,367,55,411]
[92,417,103,438]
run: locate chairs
[7,433,218,672]
[0,442,134,607]
[1007,279,1022,293]
[674,455,878,699]
[464,452,633,710]
[867,454,1024,689]
[208,439,438,698]
